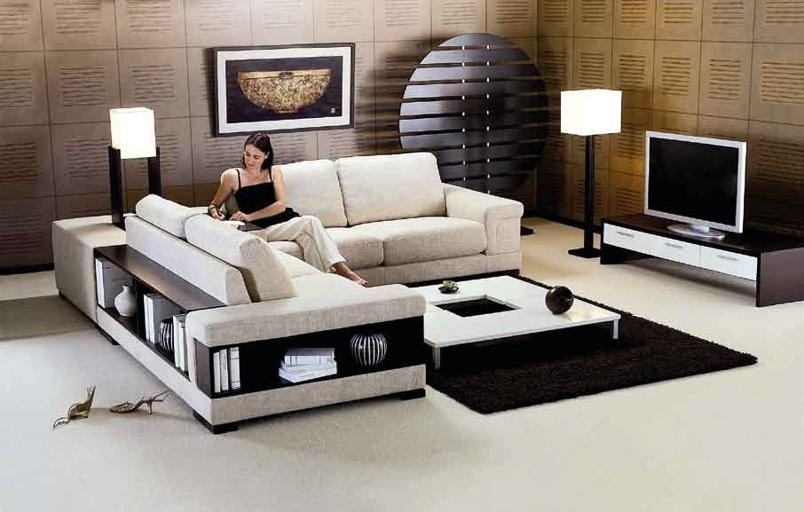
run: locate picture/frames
[211,41,356,138]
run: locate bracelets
[209,206,220,211]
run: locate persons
[208,131,369,289]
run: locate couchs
[49,207,429,436]
[266,177,525,288]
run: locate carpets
[360,273,759,416]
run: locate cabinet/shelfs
[599,213,803,308]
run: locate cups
[442,279,457,291]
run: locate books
[142,292,186,374]
[277,347,338,384]
[92,256,134,309]
[211,346,243,393]
[220,220,245,230]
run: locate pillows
[134,192,202,240]
[184,211,301,301]
[336,152,447,228]
[220,158,348,229]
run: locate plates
[438,287,459,293]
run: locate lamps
[107,105,163,229]
[558,88,624,259]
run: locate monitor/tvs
[643,128,746,240]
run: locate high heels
[53,386,95,428]
[112,387,169,414]
